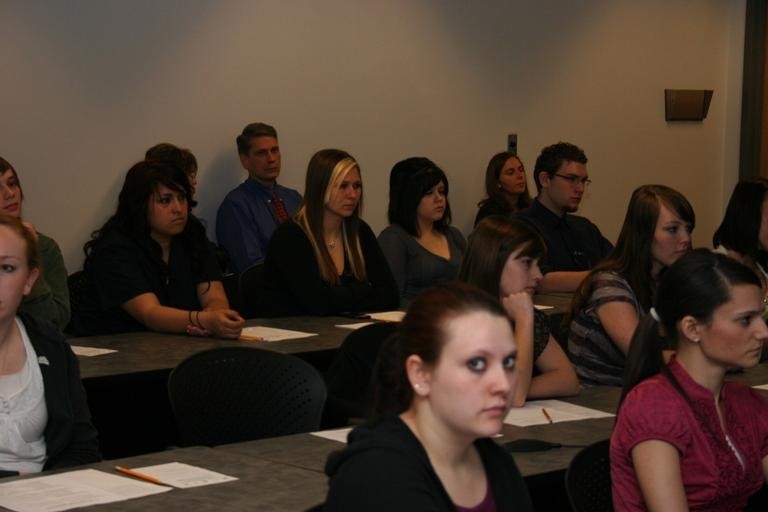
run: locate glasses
[555,173,592,185]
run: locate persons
[82,121,768,450]
[0,220,102,480]
[0,155,74,338]
[323,281,542,511]
[606,244,767,511]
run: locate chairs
[321,319,413,429]
[165,345,330,449]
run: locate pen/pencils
[115,466,171,487]
[240,335,263,340]
[542,408,552,424]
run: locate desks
[62,291,578,380]
[0,362,768,512]
